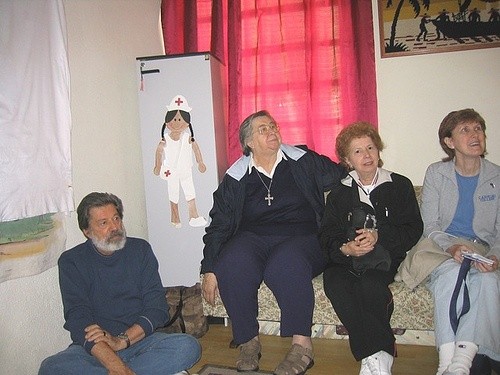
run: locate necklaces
[257,170,273,206]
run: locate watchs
[117,332,130,349]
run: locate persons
[319,121,424,375]
[200,110,354,375]
[394,108,500,375]
[37,192,201,375]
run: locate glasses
[252,124,280,135]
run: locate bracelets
[339,242,350,257]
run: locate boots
[437,342,455,375]
[443,341,478,375]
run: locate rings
[103,332,106,336]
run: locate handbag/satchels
[347,204,392,273]
[155,282,209,338]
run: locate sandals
[272,343,314,375]
[236,337,262,372]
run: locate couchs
[202,186,438,345]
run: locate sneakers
[359,358,372,375]
[365,349,393,375]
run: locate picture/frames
[377,0,500,59]
